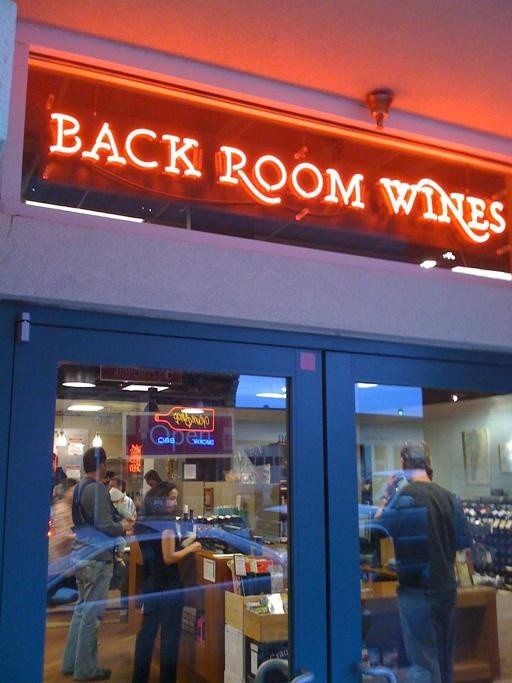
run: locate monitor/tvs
[355,383,424,418]
[234,375,290,409]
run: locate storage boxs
[224,590,289,682]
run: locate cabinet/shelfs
[357,579,500,683]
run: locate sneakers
[72,667,112,680]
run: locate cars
[46,521,288,615]
[358,469,431,555]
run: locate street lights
[91,433,102,524]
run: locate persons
[56,447,138,683]
[366,438,476,681]
[47,451,166,595]
[134,480,205,683]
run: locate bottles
[181,509,194,535]
[194,514,245,534]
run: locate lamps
[144,388,160,412]
[57,411,67,447]
[181,387,205,414]
[92,412,103,447]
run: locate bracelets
[379,494,391,503]
